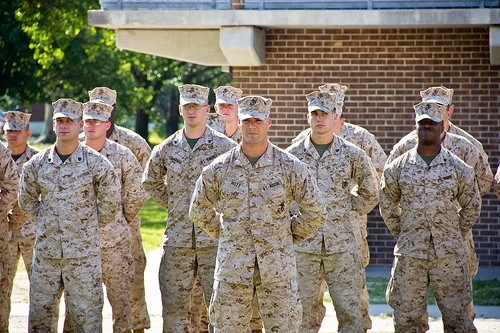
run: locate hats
[178,84,210,106]
[82,100,114,121]
[2,111,32,130]
[51,98,83,120]
[414,102,444,123]
[236,96,272,120]
[88,87,117,106]
[318,83,347,102]
[306,91,336,114]
[419,86,454,105]
[213,86,243,105]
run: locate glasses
[184,104,203,110]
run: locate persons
[199,114,262,333]
[291,83,388,331]
[4,110,39,333]
[90,86,152,333]
[18,98,122,333]
[142,84,239,333]
[380,102,482,333]
[385,86,493,321]
[284,92,381,333]
[190,96,326,333]
[62,100,143,333]
[493,165,500,198]
[211,86,244,143]
[0,141,20,264]
